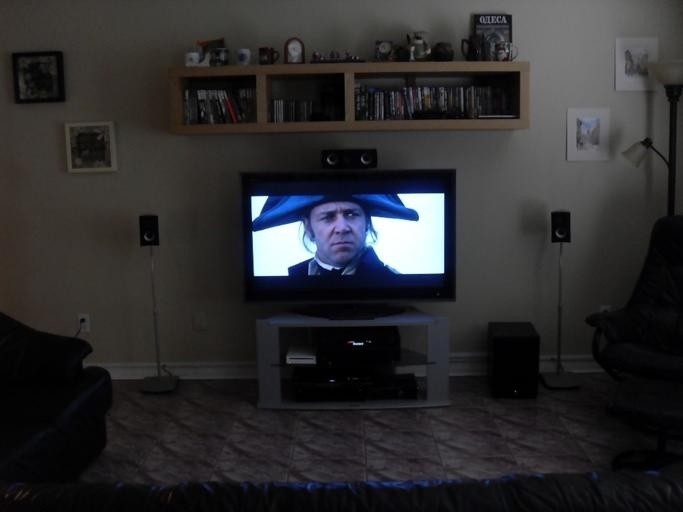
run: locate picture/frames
[63,121,119,174]
[615,38,658,91]
[567,106,610,161]
[12,51,66,103]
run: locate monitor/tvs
[234,166,460,321]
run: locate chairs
[585,214,682,469]
[1,310,114,483]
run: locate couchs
[1,469,683,512]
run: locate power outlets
[78,313,90,333]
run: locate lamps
[620,61,683,215]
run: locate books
[355,82,515,119]
[186,85,250,126]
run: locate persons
[251,192,420,289]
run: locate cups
[259,47,280,65]
[213,47,229,67]
[234,49,251,65]
[496,40,519,61]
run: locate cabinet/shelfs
[174,60,530,135]
[255,310,451,410]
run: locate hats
[251,194,420,234]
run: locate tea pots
[401,30,431,61]
[460,36,485,62]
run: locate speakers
[349,147,380,167]
[549,208,574,246]
[488,323,540,399]
[319,147,347,166]
[138,214,160,248]
